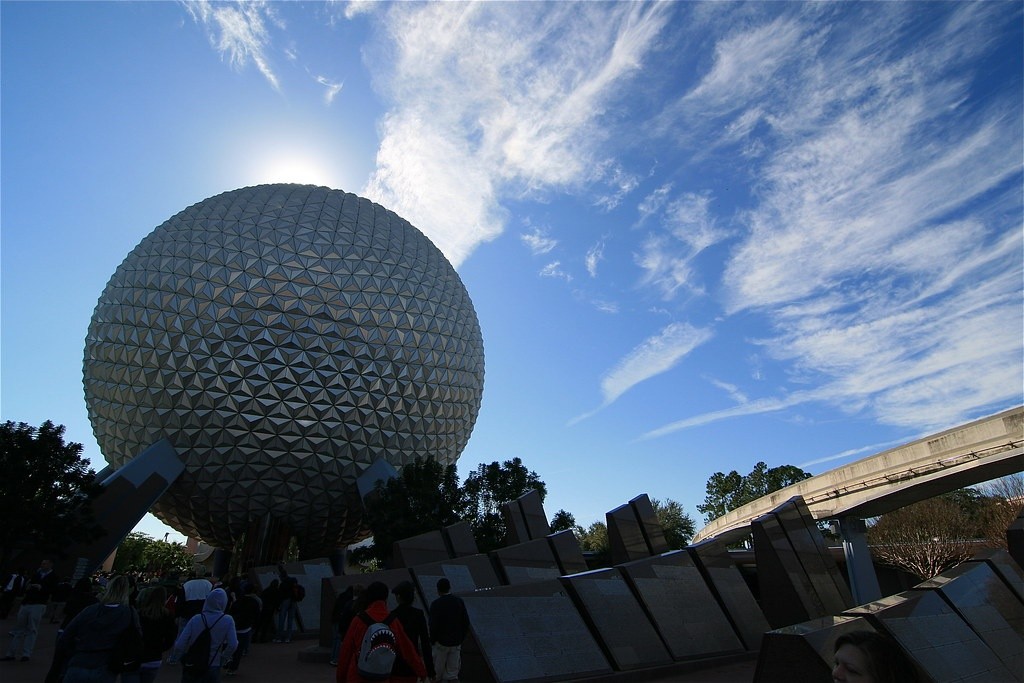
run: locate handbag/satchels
[107,606,143,674]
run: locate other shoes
[0,656,16,662]
[225,670,237,675]
[223,657,233,669]
[285,639,290,642]
[20,657,30,662]
[329,661,338,667]
[272,639,282,643]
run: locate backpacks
[356,611,398,680]
[180,612,226,683]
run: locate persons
[329,579,469,683]
[45,569,297,683]
[832,630,919,683]
[0,559,55,661]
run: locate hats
[391,581,413,594]
